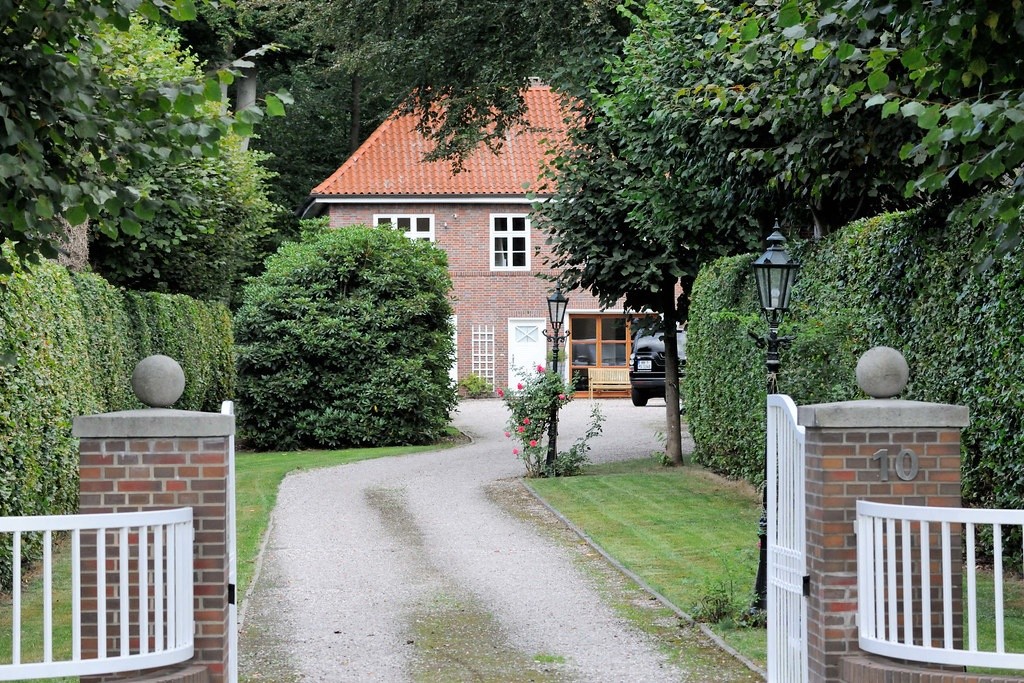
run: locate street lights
[741,215,802,630]
[540,281,570,478]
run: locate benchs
[588,367,633,400]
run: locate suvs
[629,325,689,407]
